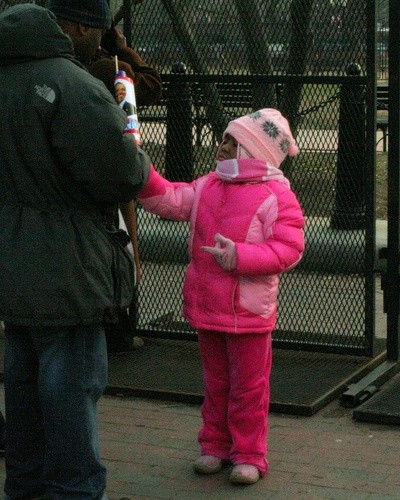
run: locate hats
[216,108,301,169]
[48,0,112,30]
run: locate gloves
[201,233,237,271]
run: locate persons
[0,0,149,500]
[114,82,137,116]
[136,108,305,484]
[86,26,162,358]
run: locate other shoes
[125,337,144,351]
[192,455,224,473]
[229,464,259,484]
